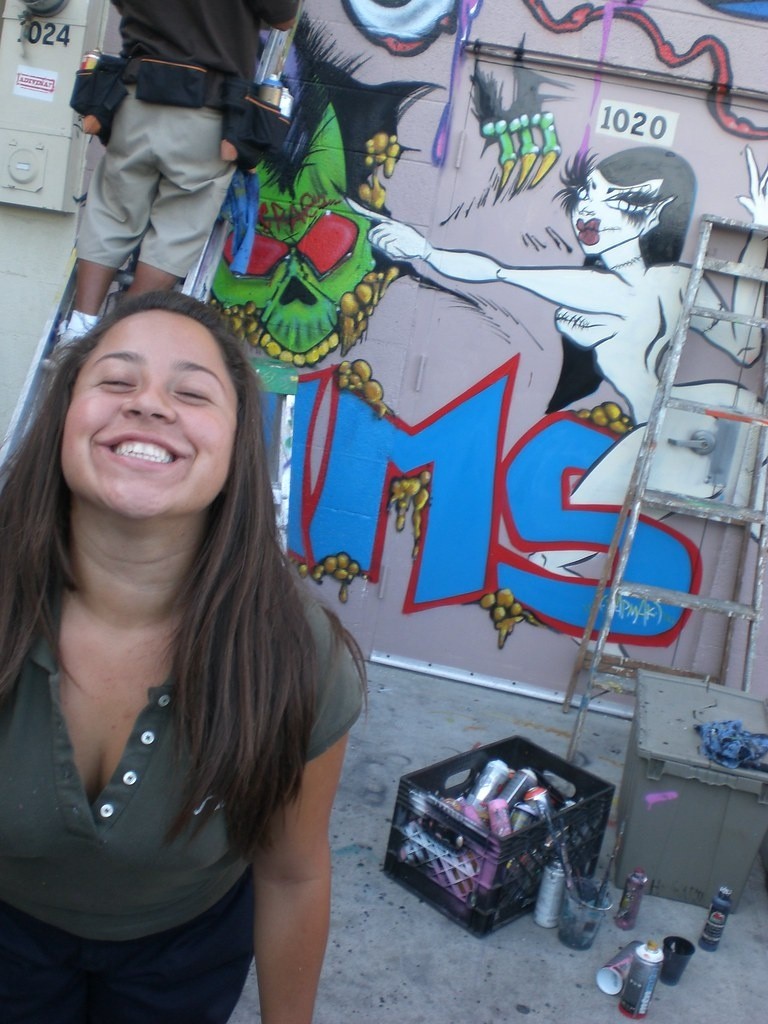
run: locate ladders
[561,211,767,767]
[0,27,290,482]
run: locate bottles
[457,797,487,829]
[488,799,513,838]
[524,787,553,820]
[534,841,572,928]
[467,759,511,806]
[617,866,648,929]
[279,88,295,117]
[511,801,540,833]
[496,766,537,809]
[260,74,283,106]
[78,48,102,75]
[698,887,733,951]
[618,939,664,1020]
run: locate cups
[660,936,696,986]
[596,940,645,995]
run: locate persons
[0,292,366,1024]
[49,0,299,359]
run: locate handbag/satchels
[70,52,130,145]
[222,75,292,172]
[136,54,207,108]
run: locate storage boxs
[615,667,768,914]
[383,734,614,940]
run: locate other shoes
[49,337,84,366]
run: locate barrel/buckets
[558,878,614,951]
